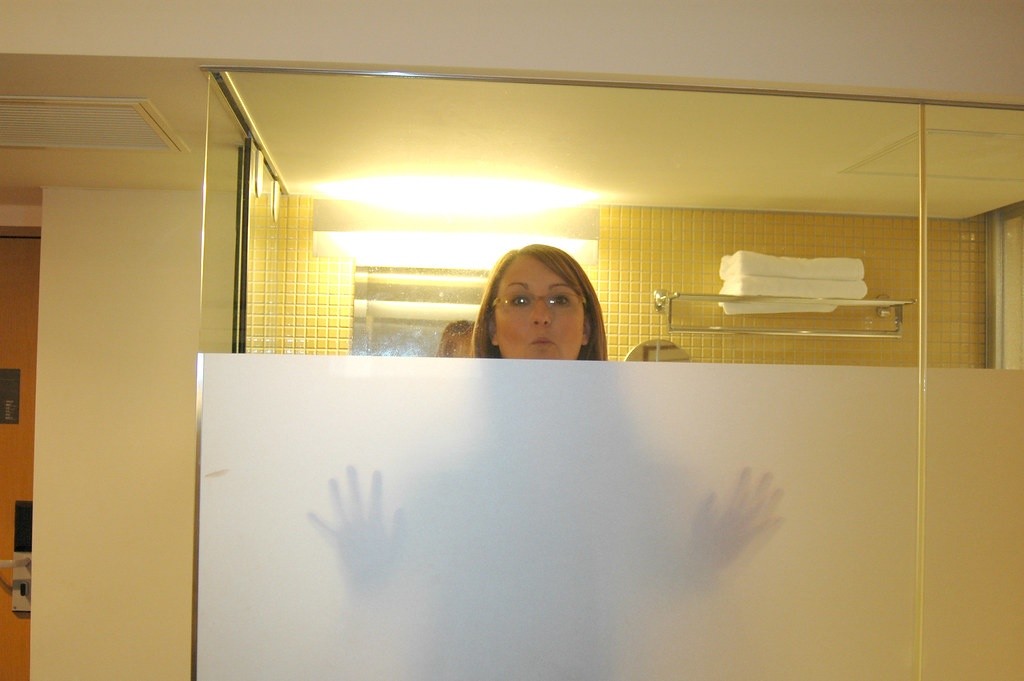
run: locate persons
[309,242,785,681]
[435,320,475,356]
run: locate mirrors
[199,63,1023,373]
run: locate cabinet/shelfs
[654,285,914,338]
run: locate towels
[718,250,868,316]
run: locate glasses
[492,291,587,314]
